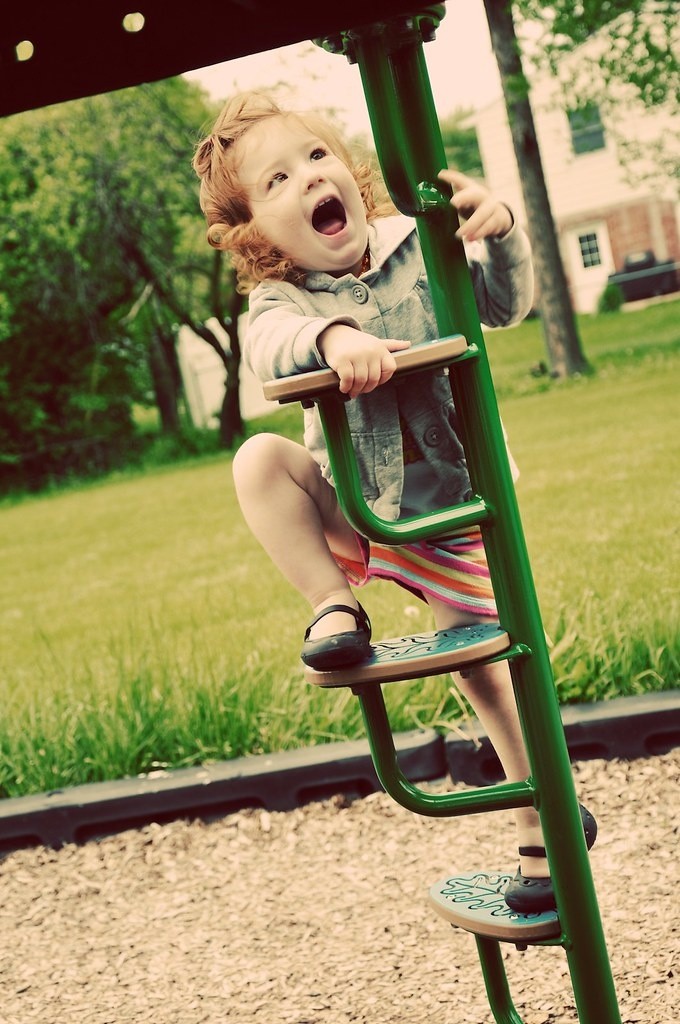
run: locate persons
[192,100,596,913]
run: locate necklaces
[359,250,368,277]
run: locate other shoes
[301,599,372,671]
[505,802,597,913]
